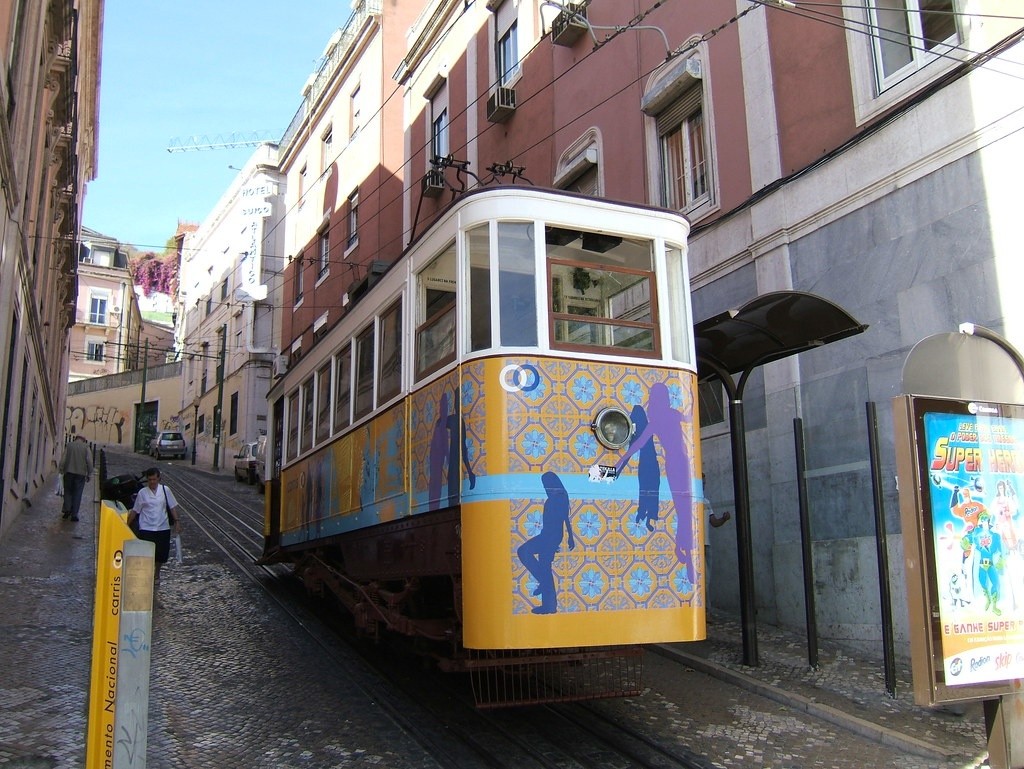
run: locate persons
[128,468,181,585]
[59,434,93,521]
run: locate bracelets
[175,519,180,521]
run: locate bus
[249,184,708,715]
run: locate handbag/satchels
[163,485,175,525]
[54,471,64,497]
[175,533,182,563]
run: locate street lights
[191,395,201,465]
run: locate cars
[148,430,188,461]
[254,428,266,495]
[233,441,258,485]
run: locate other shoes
[62,511,70,519]
[154,576,160,584]
[71,517,79,521]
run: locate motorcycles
[106,471,148,510]
